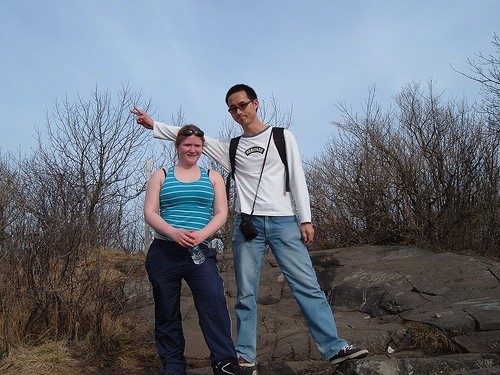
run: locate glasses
[228,101,251,114]
[181,129,204,137]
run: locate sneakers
[330,345,369,366]
[212,361,257,375]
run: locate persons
[130,84,368,367]
[144,124,243,375]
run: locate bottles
[188,245,205,265]
